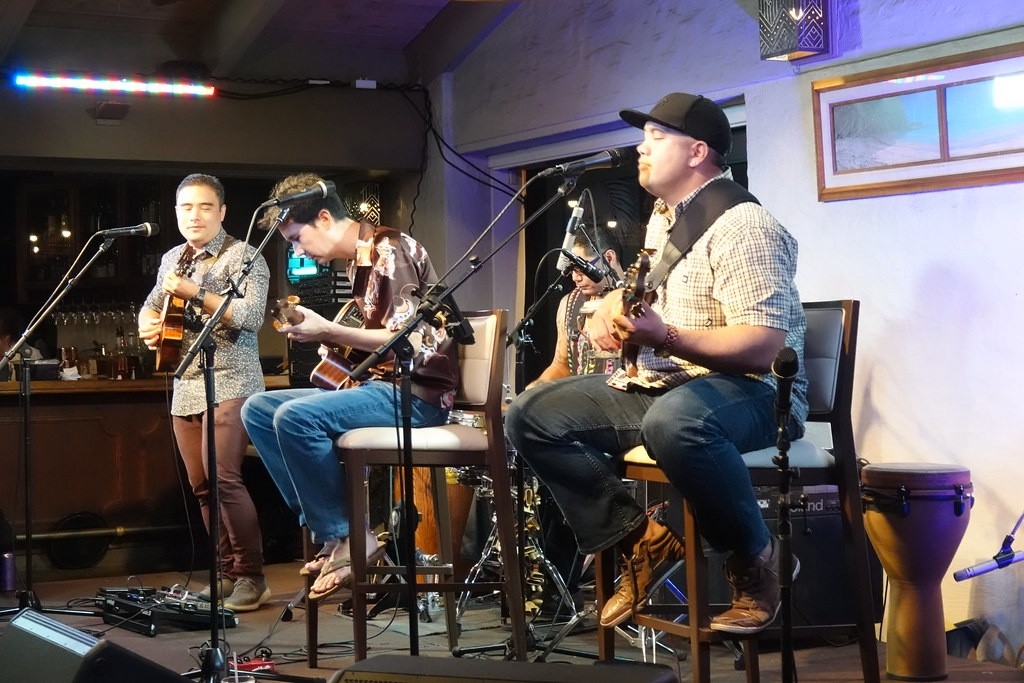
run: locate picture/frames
[810,40,1024,203]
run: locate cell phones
[427,283,475,345]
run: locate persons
[523,225,627,389]
[0,313,43,383]
[505,92,810,633]
[240,173,459,600]
[138,173,270,611]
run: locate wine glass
[47,297,143,328]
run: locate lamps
[758,0,830,61]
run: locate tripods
[451,264,746,671]
[174,204,327,683]
[0,236,114,617]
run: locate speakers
[0,608,197,683]
[327,655,680,683]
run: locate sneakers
[223,577,271,611]
[600,523,685,627]
[710,535,801,632]
[199,577,236,606]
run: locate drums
[448,409,507,487]
[395,466,473,597]
[857,463,974,681]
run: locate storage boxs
[13,364,60,381]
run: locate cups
[221,676,256,683]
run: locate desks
[0,377,300,583]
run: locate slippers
[300,539,386,575]
[308,543,385,600]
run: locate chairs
[596,300,882,683]
[303,309,531,668]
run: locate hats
[620,92,733,159]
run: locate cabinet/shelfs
[0,154,290,312]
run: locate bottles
[60,329,141,380]
[93,200,162,277]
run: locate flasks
[0,551,17,591]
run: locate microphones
[954,550,1024,582]
[96,222,159,238]
[537,147,632,179]
[560,248,604,283]
[262,181,336,208]
[771,347,801,426]
[556,191,586,270]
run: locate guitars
[270,296,365,392]
[616,249,653,378]
[155,263,196,373]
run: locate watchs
[653,324,679,359]
[191,286,205,308]
